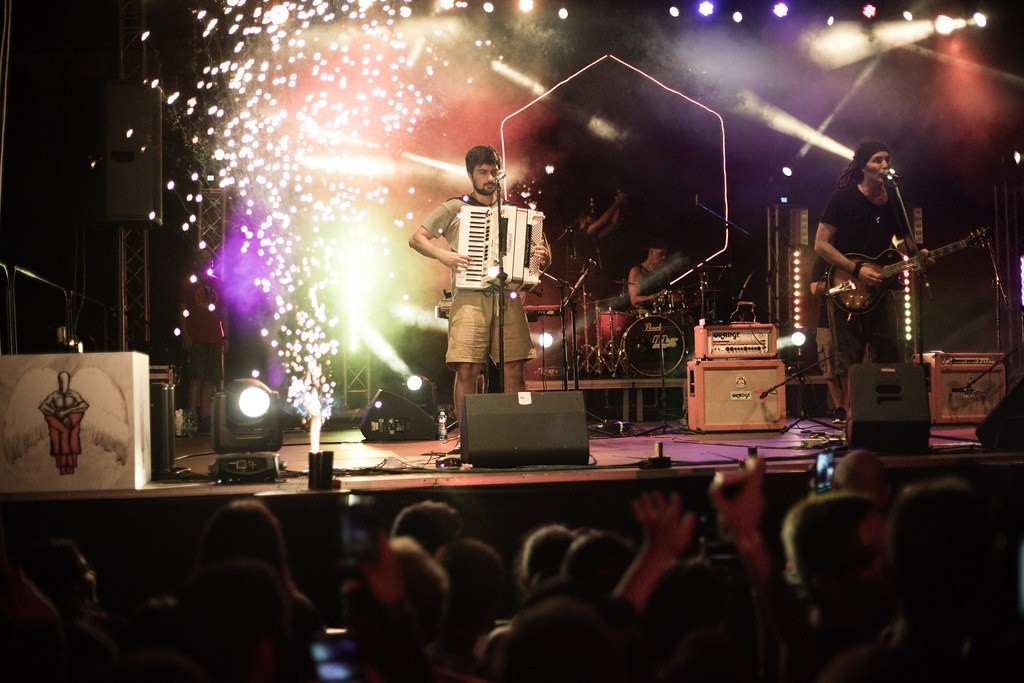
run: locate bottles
[437,408,447,440]
[176,409,198,436]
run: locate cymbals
[685,261,728,272]
[612,280,638,286]
[691,287,720,294]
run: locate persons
[628,238,679,308]
[0,447,1024,683]
[409,146,551,429]
[810,141,934,423]
[572,193,627,293]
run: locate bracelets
[852,263,864,278]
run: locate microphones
[496,171,506,181]
[880,170,903,182]
[591,198,595,213]
[952,386,974,392]
[759,388,773,399]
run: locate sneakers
[832,407,847,424]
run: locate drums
[596,310,639,355]
[658,290,688,314]
[621,314,687,377]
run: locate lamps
[210,377,283,451]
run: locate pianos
[435,299,565,322]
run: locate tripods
[573,281,630,380]
[767,352,844,434]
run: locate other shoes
[445,440,461,458]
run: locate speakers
[686,360,786,433]
[914,353,1006,423]
[88,81,163,229]
[975,377,1024,451]
[358,390,438,442]
[846,363,931,454]
[460,391,589,466]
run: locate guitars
[827,226,994,316]
